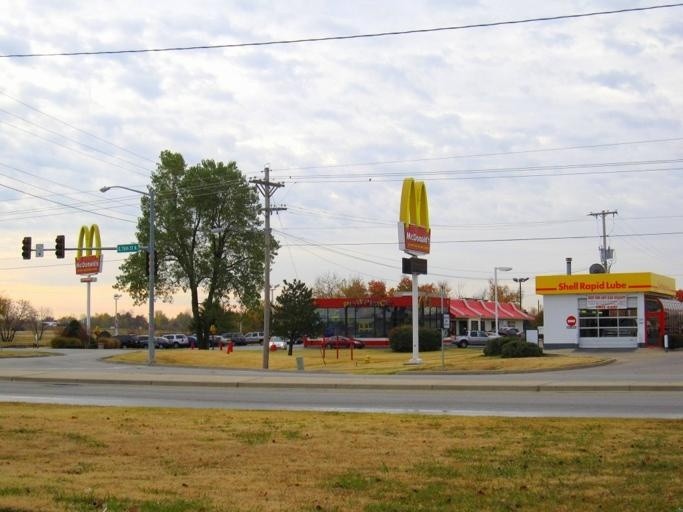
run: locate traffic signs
[35,243,44,257]
[117,244,139,253]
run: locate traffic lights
[21,236,29,260]
[54,234,64,259]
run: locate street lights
[493,265,511,341]
[98,183,155,366]
[513,276,530,313]
[112,293,122,335]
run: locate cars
[321,333,365,349]
[452,326,524,348]
[110,330,301,352]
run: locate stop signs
[565,315,575,326]
